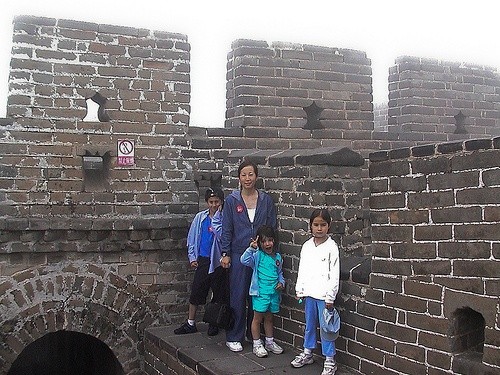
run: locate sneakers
[174,318,197,334]
[321,359,338,375]
[290,353,314,367]
[253,343,269,357]
[208,324,219,336]
[265,342,284,354]
[225,341,243,351]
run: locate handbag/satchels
[201,302,232,329]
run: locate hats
[320,307,340,341]
[205,187,224,202]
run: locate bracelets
[222,253,226,256]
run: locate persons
[290,208,340,375]
[174,188,224,336]
[240,225,285,357]
[219,161,275,353]
